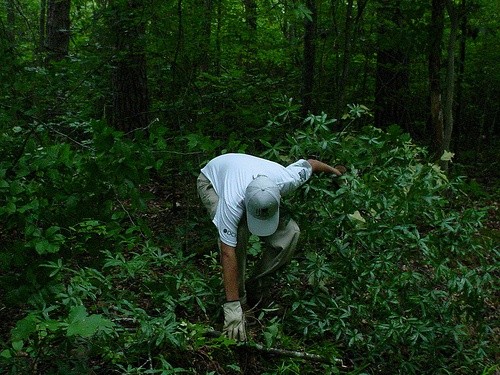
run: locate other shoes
[238,297,251,314]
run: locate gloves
[222,300,247,341]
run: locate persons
[196,151,343,343]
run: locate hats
[243,176,281,236]
